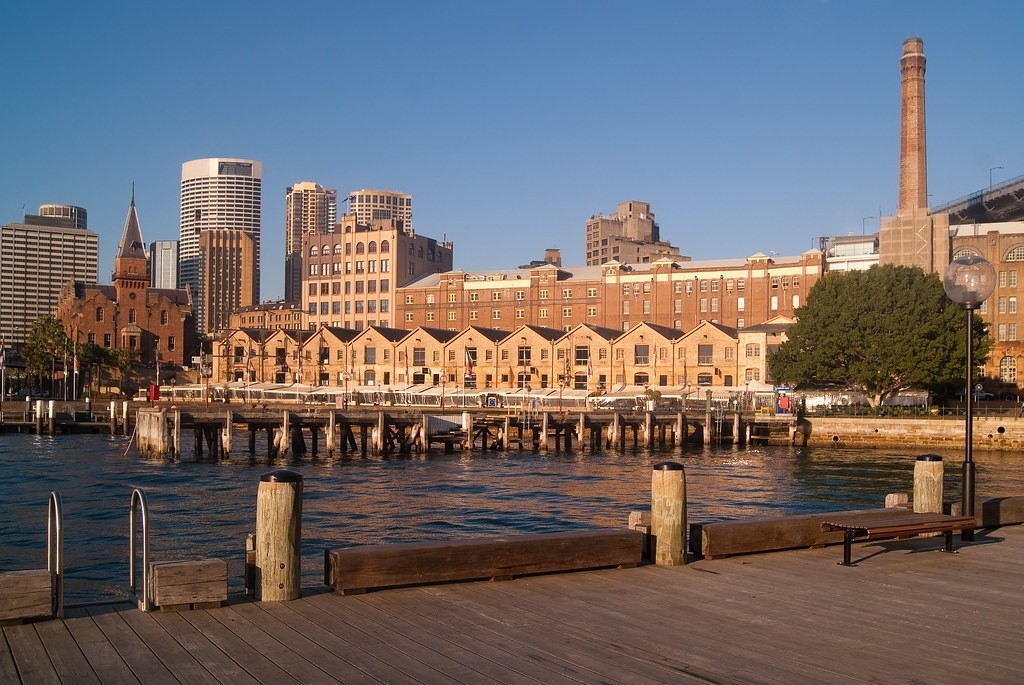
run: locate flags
[463,346,473,375]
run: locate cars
[954,388,995,401]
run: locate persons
[224,392,230,407]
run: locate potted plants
[770,411,775,418]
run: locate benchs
[820,515,980,568]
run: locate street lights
[687,381,692,413]
[439,375,448,416]
[595,383,601,411]
[341,371,351,410]
[975,384,984,412]
[744,379,750,391]
[170,378,176,404]
[942,255,998,544]
[556,378,566,415]
[695,383,702,415]
[202,367,212,409]
[643,380,650,397]
